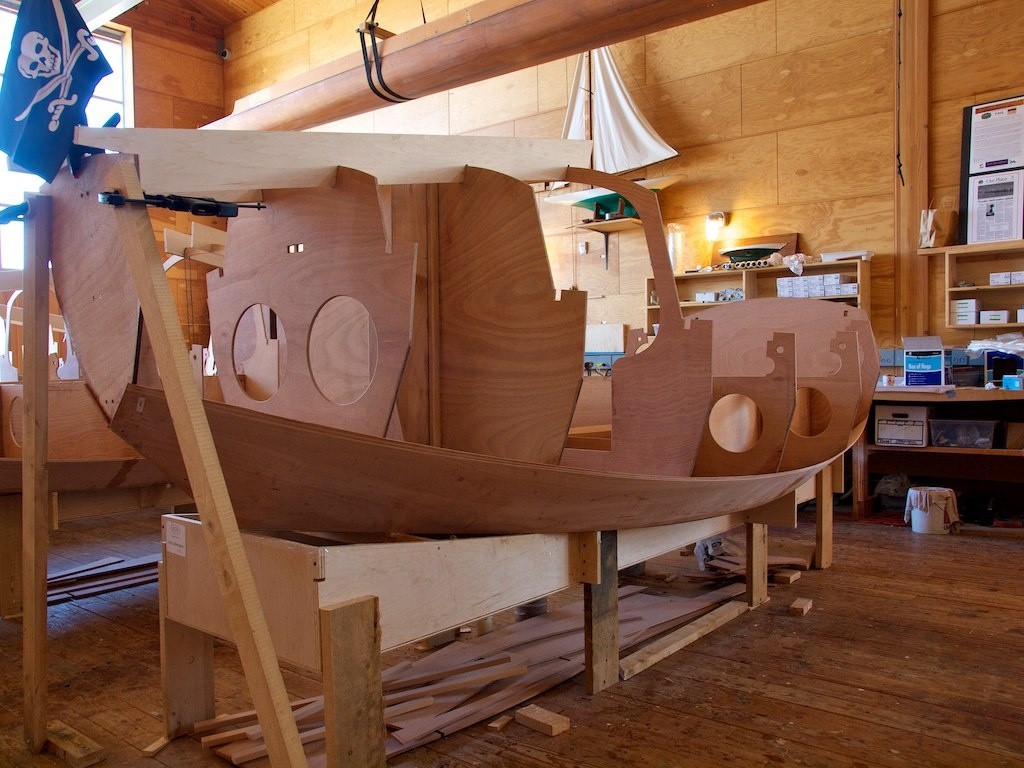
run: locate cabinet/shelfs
[644,259,871,517]
[916,239,1024,328]
[860,386,1024,512]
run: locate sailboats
[533,45,688,221]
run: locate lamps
[704,211,728,242]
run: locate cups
[882,375,895,387]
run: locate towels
[903,486,964,534]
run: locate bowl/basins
[652,324,659,335]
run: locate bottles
[650,291,659,305]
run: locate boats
[36,125,882,536]
[0,265,176,495]
[719,242,789,262]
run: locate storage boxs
[695,292,719,302]
[875,405,1024,449]
[989,271,1024,286]
[1016,308,1024,323]
[776,273,858,297]
[950,299,1011,326]
[901,336,1024,390]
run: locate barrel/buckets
[909,500,951,534]
